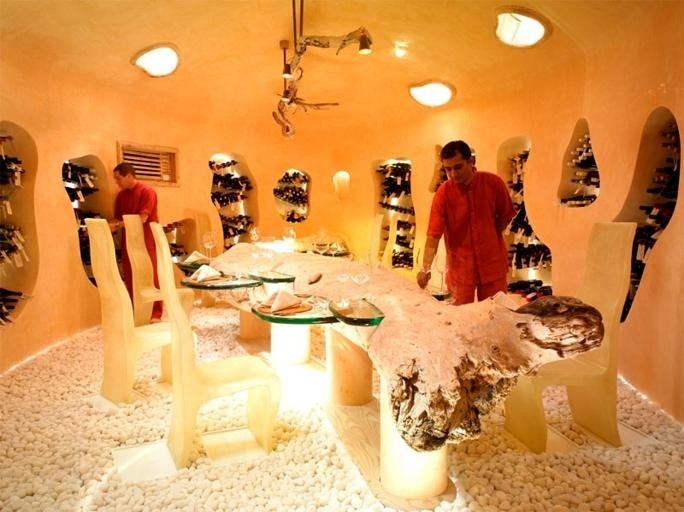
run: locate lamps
[272,0,372,138]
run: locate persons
[416,141,518,306]
[108,163,163,324]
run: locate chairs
[86,218,196,407]
[503,222,638,455]
[121,214,195,327]
[149,221,275,468]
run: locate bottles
[1,134,125,322]
[501,123,680,306]
[207,158,414,270]
